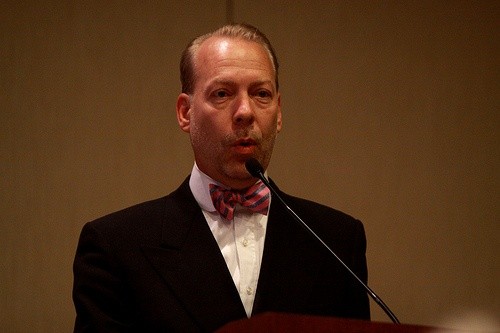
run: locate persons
[71,22,374,333]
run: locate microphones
[245,157,401,322]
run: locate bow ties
[209,180,270,223]
